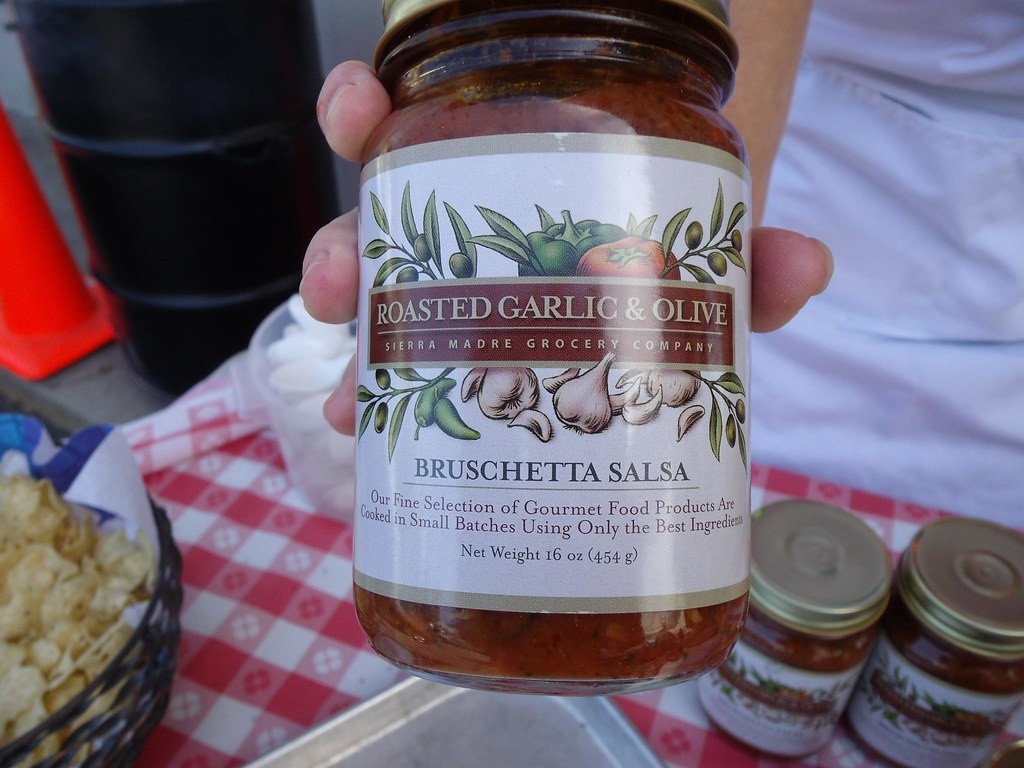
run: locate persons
[297,0,1023,537]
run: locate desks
[101,351,1024,768]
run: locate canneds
[347,0,751,691]
[693,498,1024,768]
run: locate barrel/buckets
[12,0,342,410]
